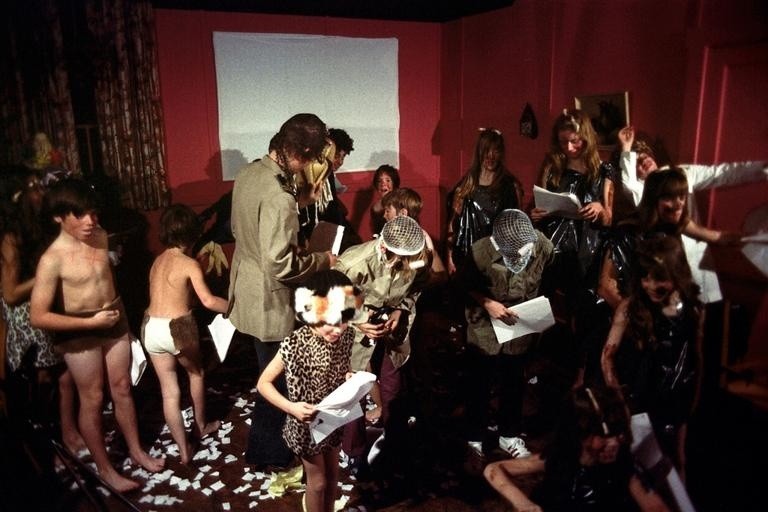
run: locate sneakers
[498,437,532,458]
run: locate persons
[139,203,231,466]
[1,177,86,466]
[27,178,166,495]
[225,107,768,512]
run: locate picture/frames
[573,91,629,152]
